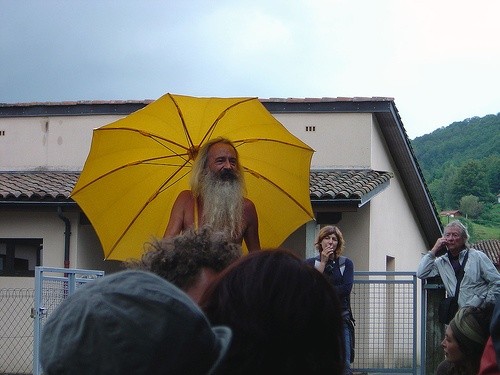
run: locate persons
[304,225,356,375]
[416,220,500,360]
[163,135,260,254]
[39,270,233,375]
[197,247,347,375]
[436,302,495,375]
[478,294,500,375]
[121,223,244,305]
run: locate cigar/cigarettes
[328,246,332,249]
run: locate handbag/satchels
[349,311,355,364]
[438,297,459,325]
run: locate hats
[449,300,495,354]
[39,269,233,375]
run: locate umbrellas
[65,92,317,263]
